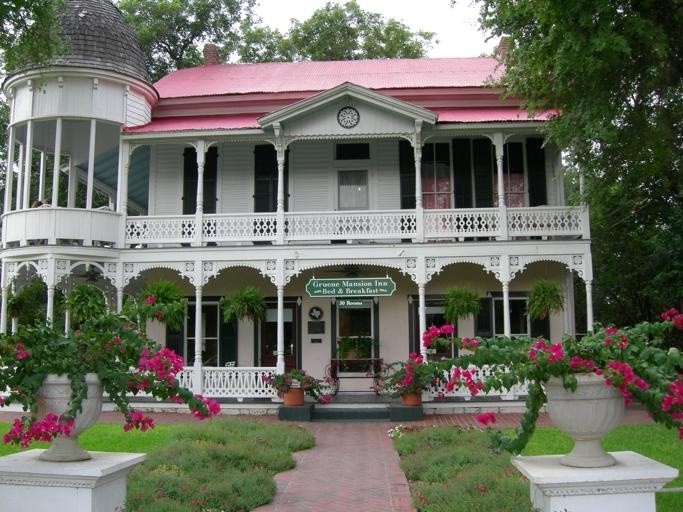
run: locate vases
[284,388,304,406]
[262,368,331,404]
[401,392,421,406]
[29,372,103,462]
[543,371,625,469]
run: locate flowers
[1,294,221,450]
[374,352,423,398]
[422,308,683,456]
